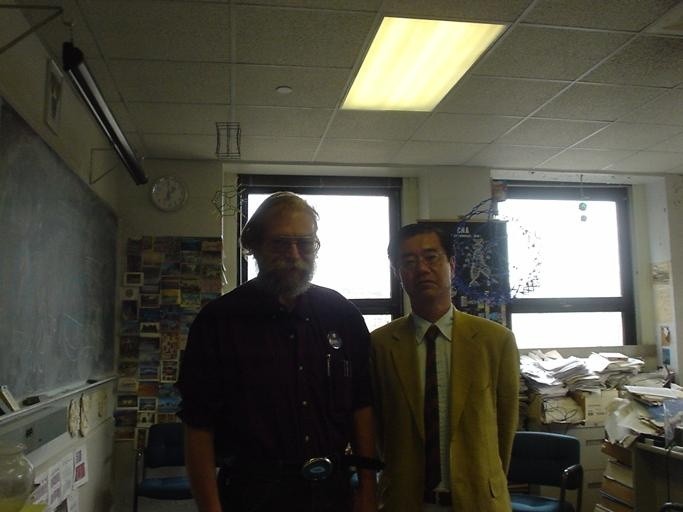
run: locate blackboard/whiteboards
[0,93,120,427]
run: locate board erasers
[23,396,40,406]
[86,379,98,384]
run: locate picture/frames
[44,58,64,138]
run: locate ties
[422,324,443,492]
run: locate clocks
[149,174,188,213]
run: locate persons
[369,223,520,512]
[175,190,376,512]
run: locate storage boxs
[593,426,640,512]
[569,389,618,427]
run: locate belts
[215,454,354,483]
[420,490,454,506]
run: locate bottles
[0,440,35,512]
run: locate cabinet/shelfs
[528,421,608,512]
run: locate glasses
[266,236,320,256]
[398,251,447,270]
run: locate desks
[633,439,683,512]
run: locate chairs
[131,421,198,512]
[659,501,683,512]
[507,431,585,512]
